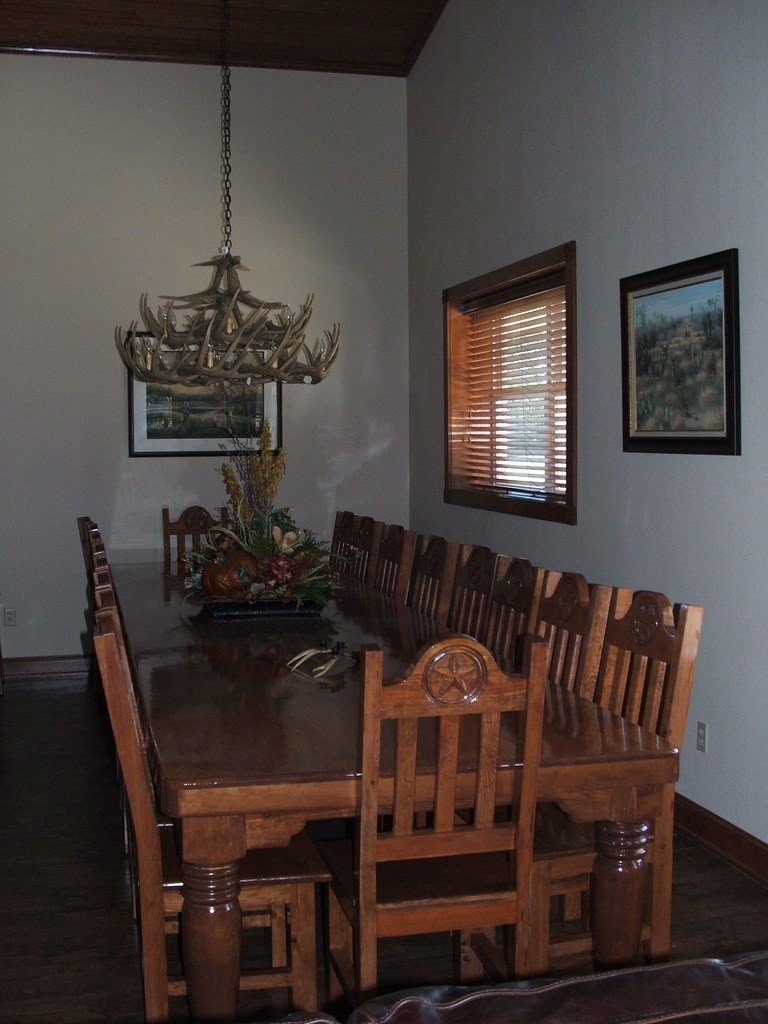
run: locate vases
[203,598,324,622]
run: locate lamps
[116,0,343,386]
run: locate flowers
[167,410,362,601]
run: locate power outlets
[4,608,16,625]
[698,720,707,753]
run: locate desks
[111,563,681,1024]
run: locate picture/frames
[126,331,283,459]
[619,248,741,457]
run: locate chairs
[313,632,548,1007]
[329,507,704,976]
[78,514,332,1024]
[163,505,228,561]
